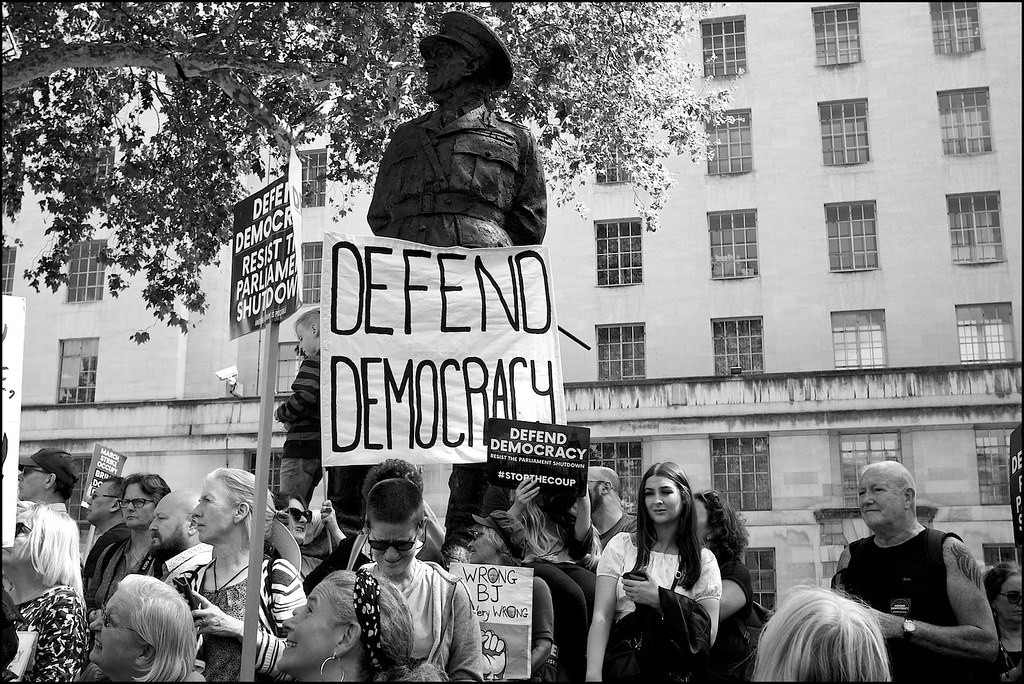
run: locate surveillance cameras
[213,364,239,381]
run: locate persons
[358,478,483,684]
[274,492,345,580]
[83,466,214,610]
[179,468,307,684]
[586,460,722,682]
[831,459,999,683]
[0,500,88,683]
[694,491,752,681]
[272,307,324,502]
[750,586,892,682]
[984,561,1024,684]
[467,511,557,683]
[588,466,638,550]
[328,10,548,552]
[277,568,445,682]
[301,458,446,600]
[88,573,208,681]
[19,445,79,506]
[512,475,600,683]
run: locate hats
[471,509,529,561]
[19,448,80,489]
[211,519,301,574]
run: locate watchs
[901,617,917,641]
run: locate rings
[202,619,205,626]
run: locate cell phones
[627,571,648,581]
[172,573,200,610]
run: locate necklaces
[999,639,1016,679]
[211,555,249,604]
[130,543,144,562]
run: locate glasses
[14,523,32,538]
[117,497,155,508]
[367,524,421,552]
[998,592,1022,604]
[285,508,312,523]
[100,602,137,632]
[473,531,484,542]
[22,466,50,477]
[92,492,120,499]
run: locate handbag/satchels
[603,635,641,683]
